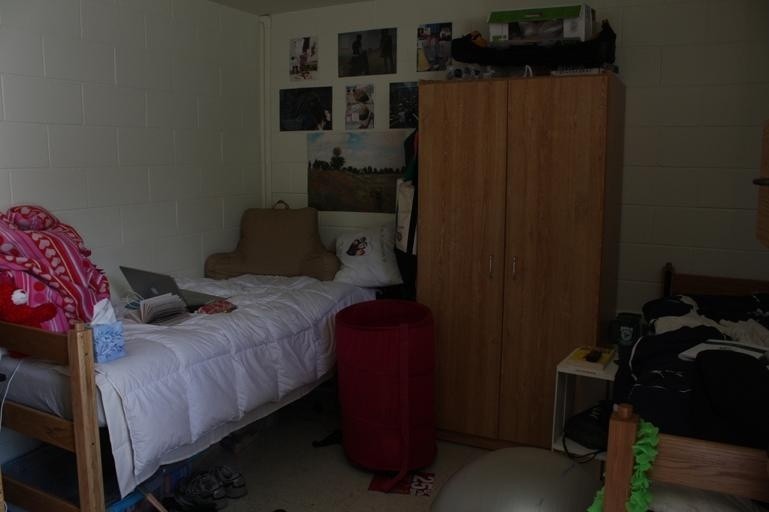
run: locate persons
[417,26,452,72]
[290,56,298,73]
[299,46,311,79]
[397,103,407,127]
[346,89,369,122]
[377,29,393,69]
[352,33,370,75]
[318,107,332,131]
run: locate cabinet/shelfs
[412,71,621,452]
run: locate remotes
[585,349,598,361]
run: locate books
[124,291,192,326]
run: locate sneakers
[177,474,228,509]
[202,464,249,499]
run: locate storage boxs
[487,5,598,43]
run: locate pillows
[333,221,405,289]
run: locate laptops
[119,265,227,313]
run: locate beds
[589,258,769,511]
[1,206,401,512]
